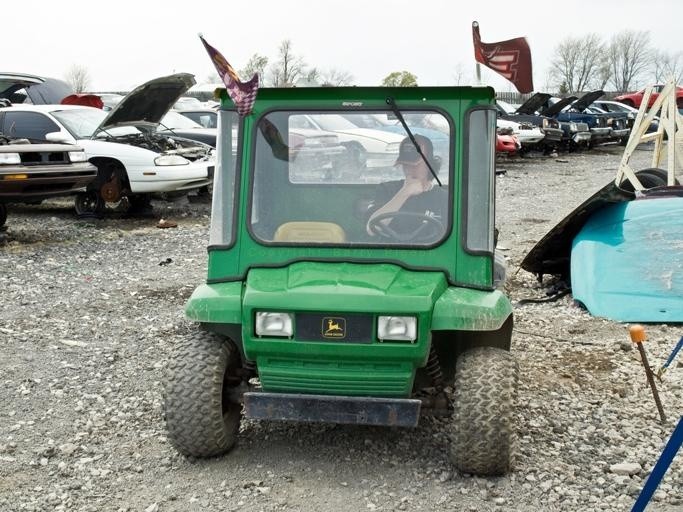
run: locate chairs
[272,220,347,244]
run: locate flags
[472,30,535,97]
[202,37,258,118]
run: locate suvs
[156,84,518,477]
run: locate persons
[360,131,448,242]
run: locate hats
[393,135,433,168]
